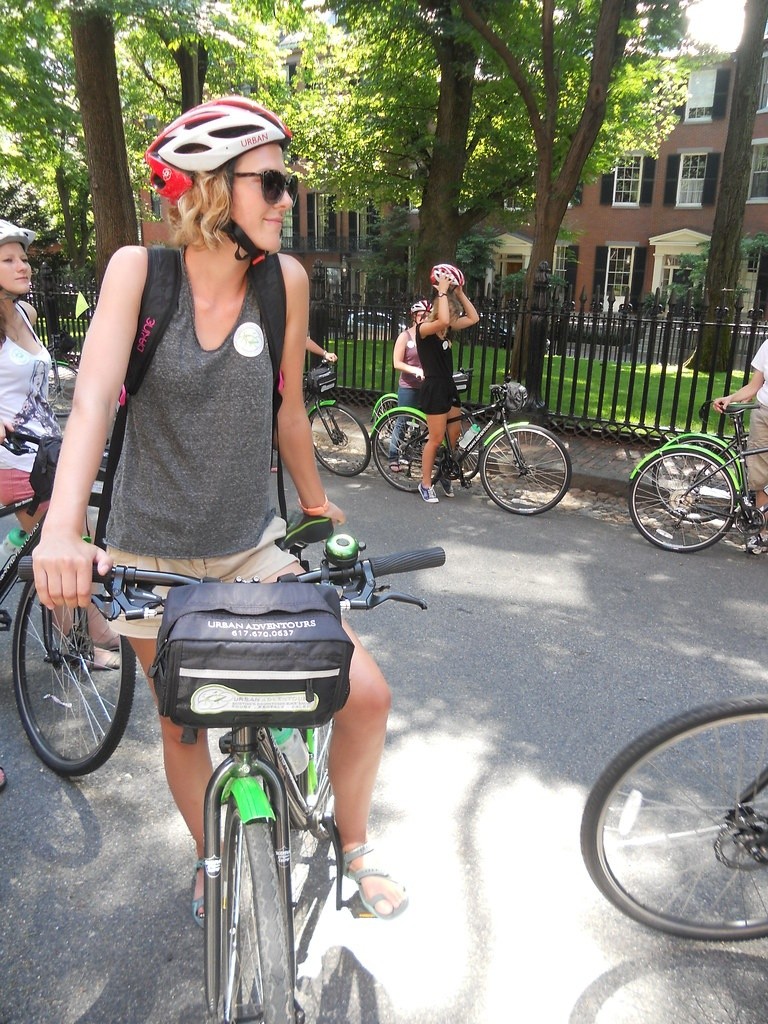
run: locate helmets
[411,300,434,315]
[0,219,37,252]
[142,96,292,202]
[430,264,465,288]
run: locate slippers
[389,465,402,473]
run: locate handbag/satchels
[451,372,470,393]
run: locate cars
[342,309,409,341]
[457,311,550,355]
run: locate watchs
[438,291,447,297]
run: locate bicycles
[368,374,573,517]
[0,424,133,776]
[627,401,767,557]
[302,355,372,478]
[19,512,445,1024]
[370,367,486,482]
[579,699,768,946]
[46,323,80,418]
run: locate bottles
[459,424,480,448]
[0,528,27,573]
[267,725,309,775]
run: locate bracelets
[322,350,327,357]
[299,494,329,516]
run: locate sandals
[341,841,408,919]
[192,859,205,929]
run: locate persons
[388,299,434,472]
[32,101,409,930]
[1,218,120,669]
[306,338,337,362]
[713,339,768,554]
[415,269,480,502]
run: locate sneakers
[440,479,455,497]
[746,531,768,554]
[418,483,440,503]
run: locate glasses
[234,169,300,207]
